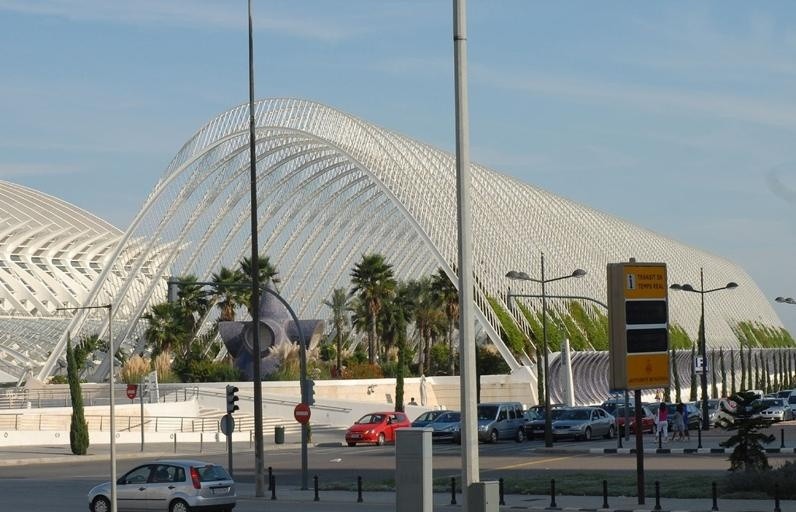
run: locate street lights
[774,297,795,304]
[669,268,739,432]
[54,303,117,512]
[504,252,586,446]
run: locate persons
[408,398,417,405]
[654,402,668,442]
[669,404,686,442]
[675,403,690,442]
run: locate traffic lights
[303,379,317,405]
[225,384,240,414]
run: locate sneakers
[654,438,690,443]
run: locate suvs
[86,457,236,512]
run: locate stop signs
[126,384,137,399]
[292,403,312,425]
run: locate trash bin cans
[275,426,284,443]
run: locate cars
[424,412,461,444]
[344,410,410,446]
[411,410,449,431]
